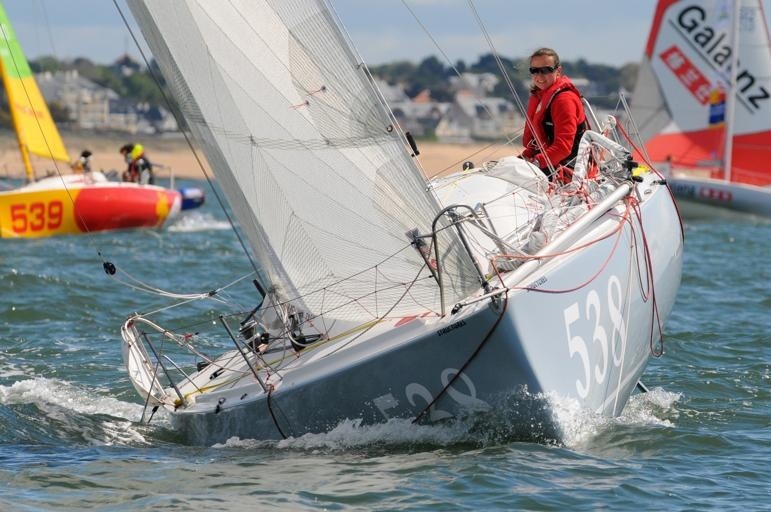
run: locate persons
[120,142,156,187]
[77,149,94,172]
[519,46,590,185]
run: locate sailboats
[0,2,206,240]
[114,0,685,455]
[617,0,769,217]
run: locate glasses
[529,66,557,74]
